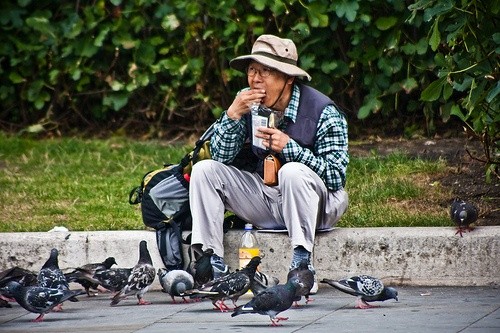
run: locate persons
[190,34,350,293]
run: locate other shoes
[212,264,229,280]
[289,262,318,294]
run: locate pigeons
[0,240,398,323]
[451,196,478,237]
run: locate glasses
[245,64,277,78]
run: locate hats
[229,34,313,81]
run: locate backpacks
[128,140,260,272]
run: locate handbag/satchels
[263,153,281,187]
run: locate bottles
[238,223,260,299]
